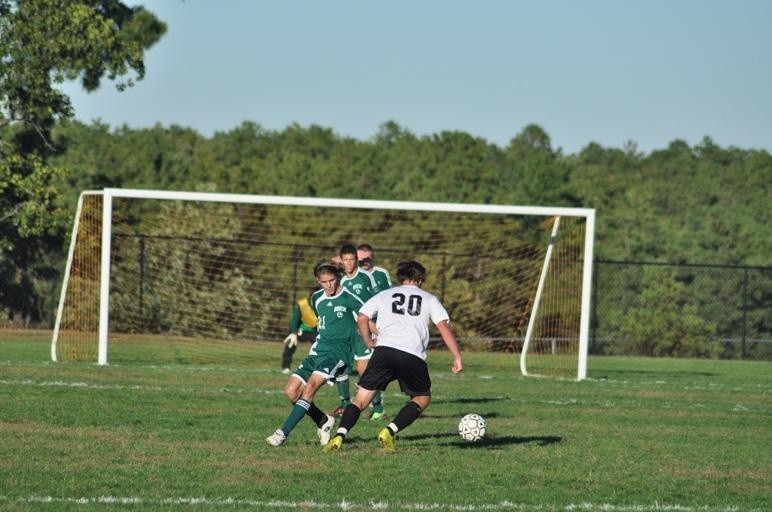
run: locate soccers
[458,414,486,443]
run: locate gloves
[283,333,298,348]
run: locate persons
[328,244,387,419]
[279,286,320,375]
[332,242,396,407]
[264,259,379,448]
[320,261,464,454]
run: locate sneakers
[379,427,396,454]
[266,428,286,446]
[370,407,385,420]
[329,406,344,417]
[318,414,336,445]
[324,436,343,453]
